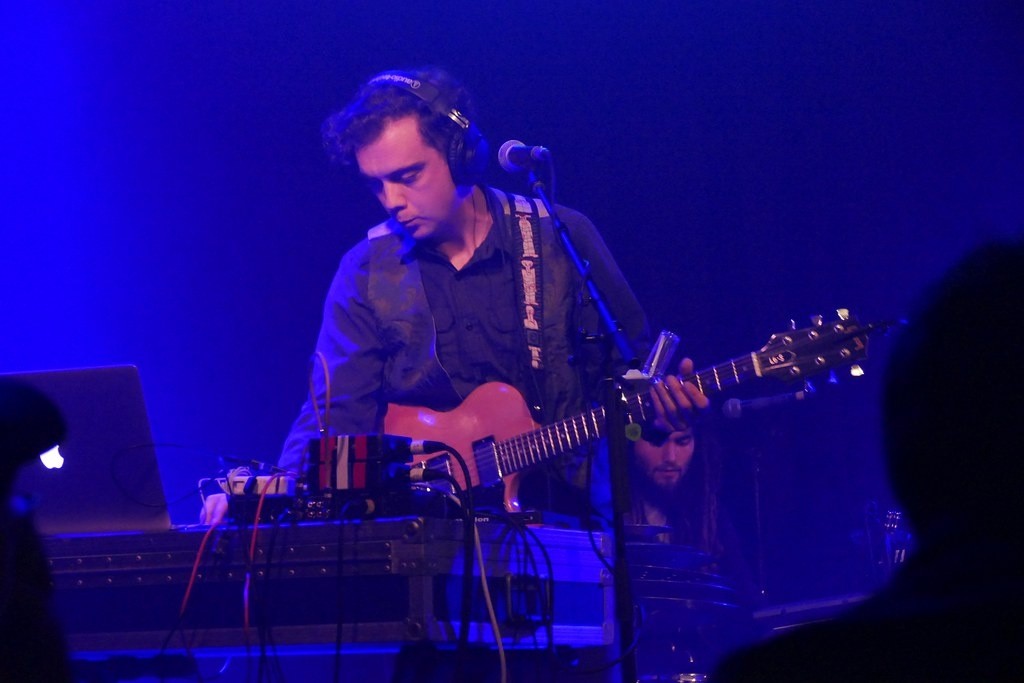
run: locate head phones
[368,69,490,187]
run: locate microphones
[724,390,810,418]
[499,140,549,174]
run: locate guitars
[383,308,909,528]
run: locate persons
[274,66,711,510]
[595,412,711,546]
[705,237,1024,683]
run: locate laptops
[0,364,221,536]
